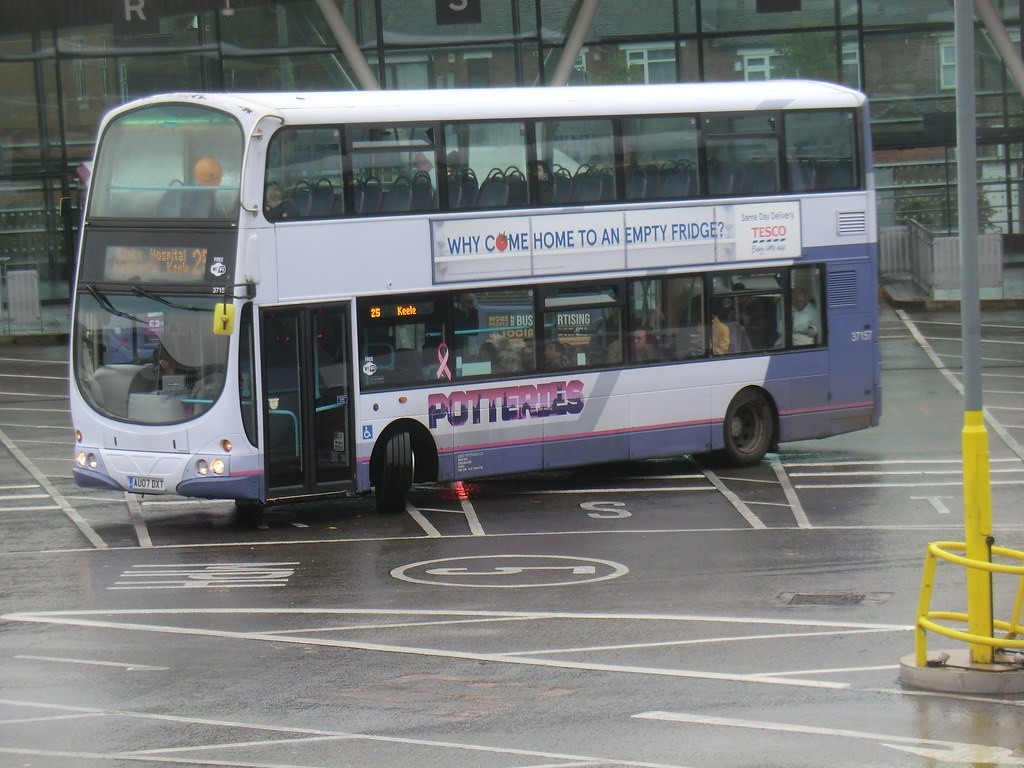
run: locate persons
[536,162,552,205]
[154,284,759,416]
[773,286,821,350]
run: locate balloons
[194,156,223,191]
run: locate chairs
[263,155,855,223]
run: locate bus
[69,78,882,514]
